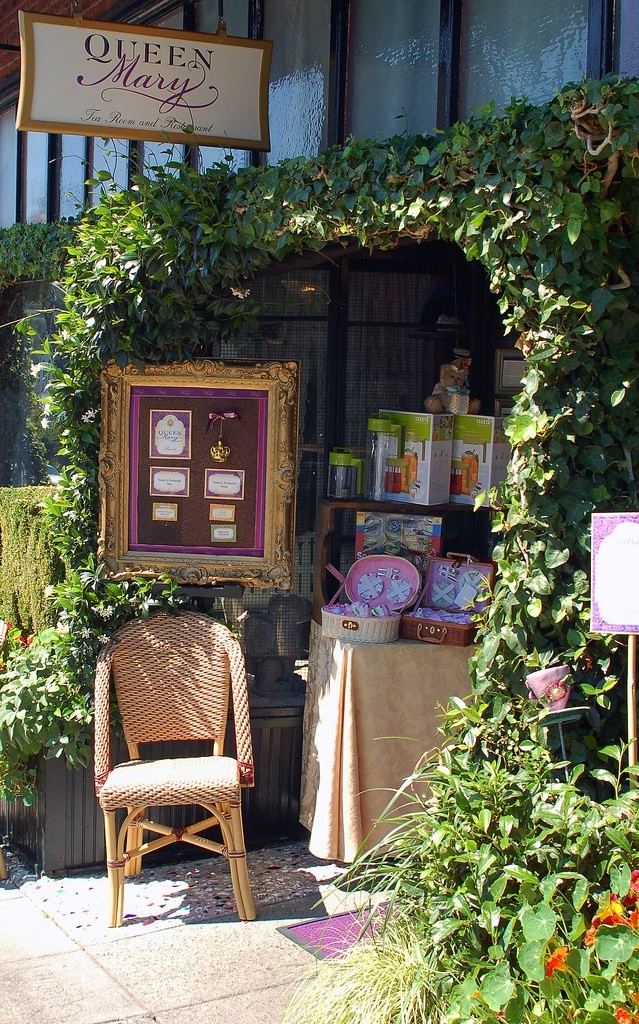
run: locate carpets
[276,899,400,961]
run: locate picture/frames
[97,357,300,591]
[494,398,514,416]
[494,349,525,394]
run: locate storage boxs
[378,408,454,506]
[451,415,511,507]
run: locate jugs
[384,458,408,493]
[449,461,469,494]
[326,446,363,500]
[364,413,402,502]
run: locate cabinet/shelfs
[297,496,497,863]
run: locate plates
[459,570,483,592]
[387,580,410,603]
[357,572,384,599]
[431,583,455,607]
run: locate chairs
[94,610,255,929]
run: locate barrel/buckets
[268,594,312,657]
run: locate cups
[525,664,572,711]
[372,604,390,618]
[354,602,372,618]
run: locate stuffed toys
[424,347,481,414]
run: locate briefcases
[402,552,495,647]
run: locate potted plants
[523,649,572,711]
